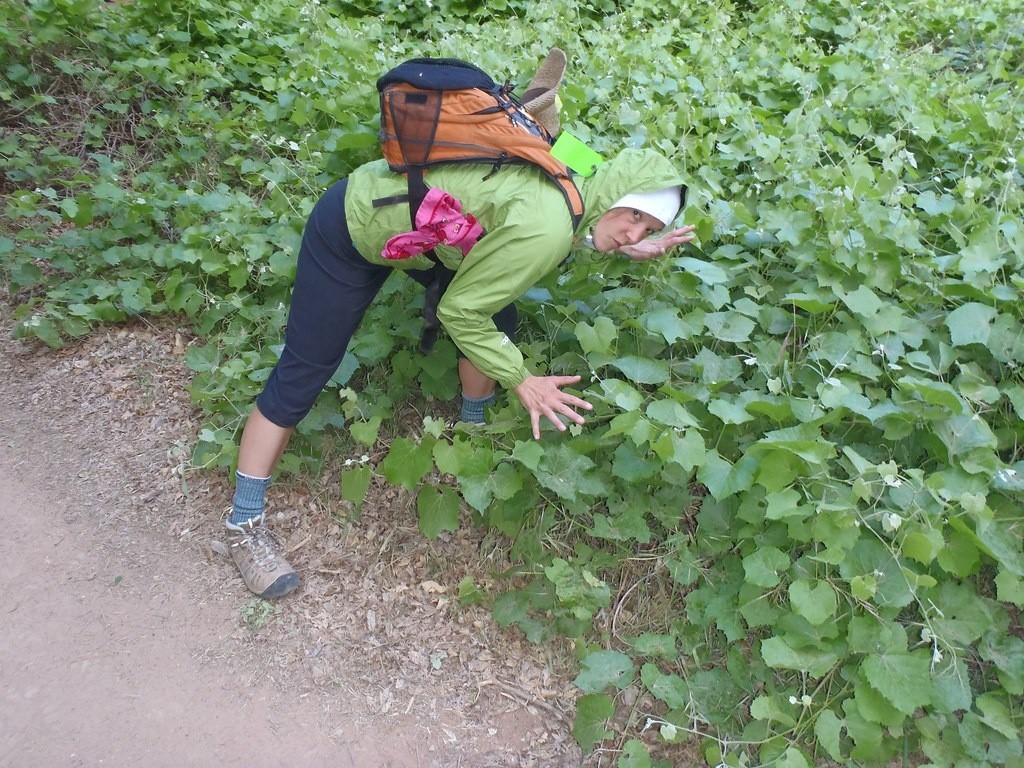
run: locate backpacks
[377,58,585,353]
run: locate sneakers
[226,512,298,601]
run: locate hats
[519,47,567,139]
[608,186,682,230]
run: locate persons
[222,148,698,600]
